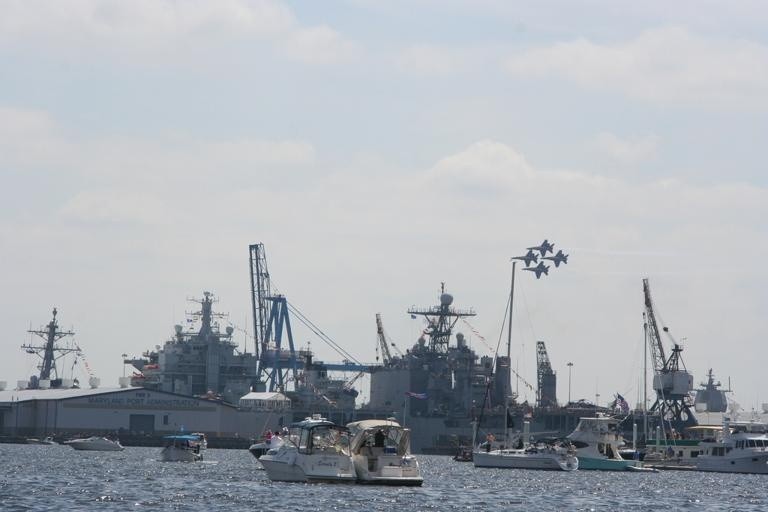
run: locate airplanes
[511,239,569,279]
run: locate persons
[486,433,495,451]
[668,445,672,456]
[633,448,640,460]
[271,431,283,448]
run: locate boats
[63,436,125,451]
[247,412,424,486]
[162,431,207,461]
[625,312,768,474]
[472,261,641,471]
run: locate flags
[616,394,630,411]
[405,391,429,400]
